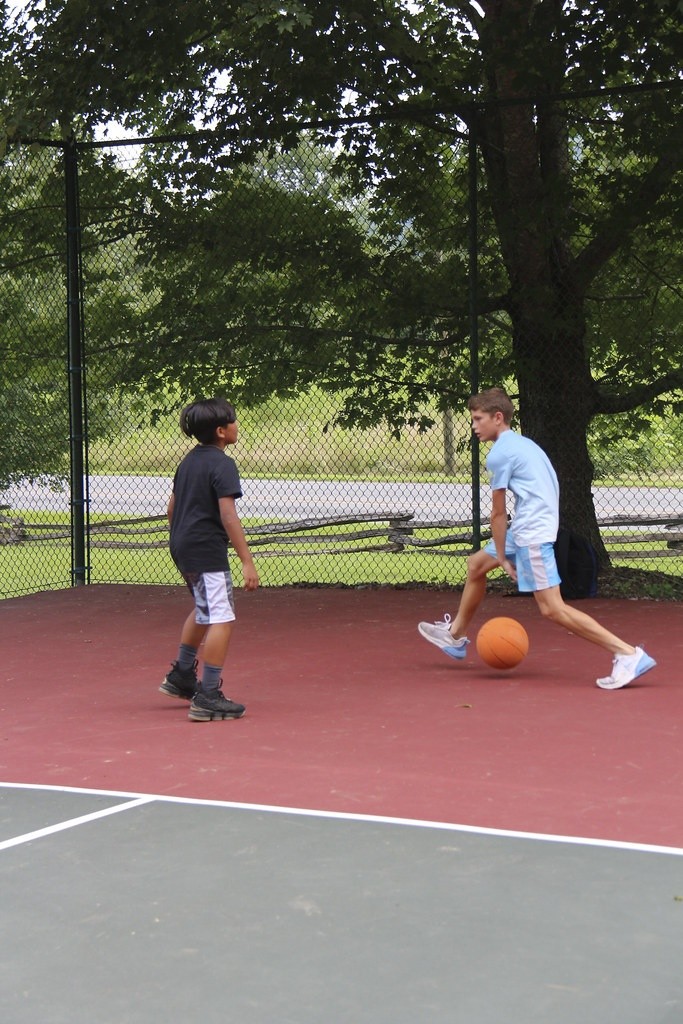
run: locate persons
[157,398,260,722]
[417,389,658,690]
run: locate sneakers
[417,620,471,660]
[188,683,246,720]
[158,661,204,700]
[595,646,658,690]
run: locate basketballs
[476,616,529,670]
